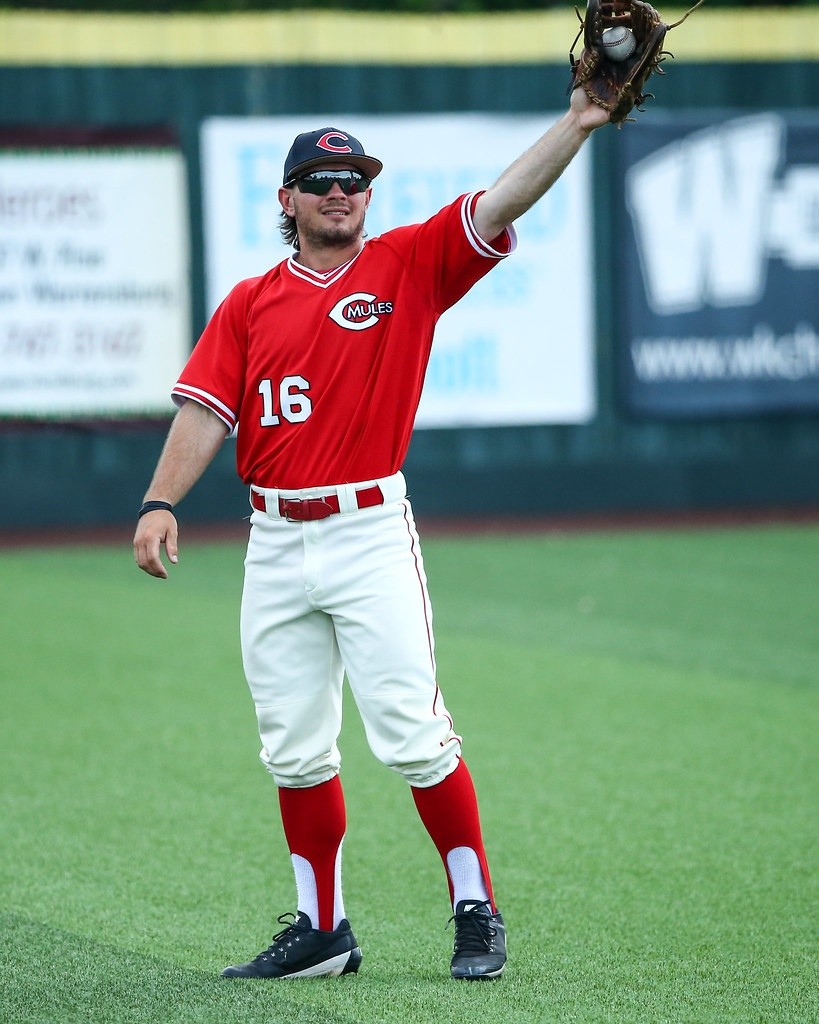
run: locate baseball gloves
[564,0,705,133]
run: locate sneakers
[444,895,507,981]
[220,910,363,981]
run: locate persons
[134,0,666,983]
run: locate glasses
[288,170,370,197]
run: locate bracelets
[139,501,173,518]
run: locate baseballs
[601,26,637,62]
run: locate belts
[248,487,383,523]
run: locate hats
[283,127,382,182]
